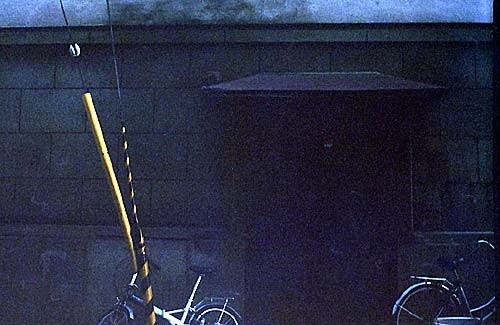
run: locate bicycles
[392,238,499,324]
[95,258,243,324]
